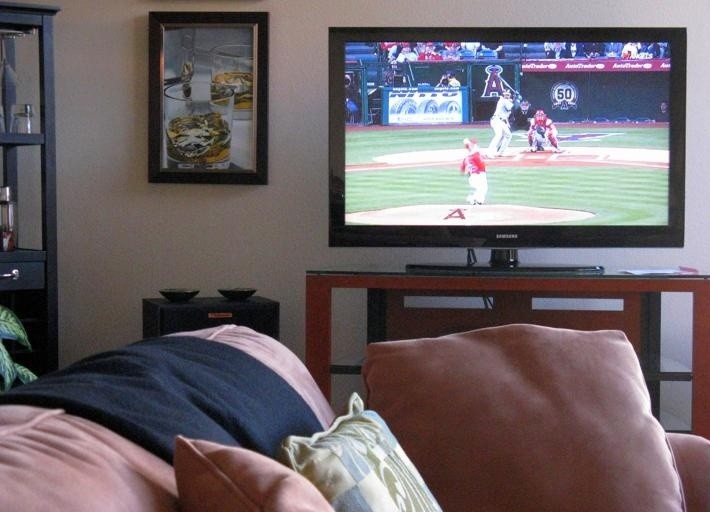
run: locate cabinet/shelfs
[0,0,62,386]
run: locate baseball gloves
[536,126,545,134]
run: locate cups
[206,42,253,121]
[163,80,235,170]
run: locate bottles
[0,185,18,252]
[9,104,34,134]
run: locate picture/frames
[147,10,270,185]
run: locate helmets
[536,110,545,121]
[520,100,530,110]
[502,89,510,99]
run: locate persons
[462,137,488,205]
[488,88,565,157]
[380,40,505,63]
[544,39,669,60]
[435,71,461,87]
[656,101,670,122]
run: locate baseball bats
[498,75,516,93]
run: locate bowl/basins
[158,287,200,303]
[216,287,257,301]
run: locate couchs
[0,322,709,511]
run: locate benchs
[378,41,670,62]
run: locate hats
[465,139,477,148]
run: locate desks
[379,86,473,126]
[303,268,710,441]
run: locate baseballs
[520,72,523,76]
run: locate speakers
[393,74,403,87]
[142,297,280,341]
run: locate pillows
[173,432,334,511]
[280,391,446,511]
[361,322,687,512]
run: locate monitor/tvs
[327,26,687,276]
